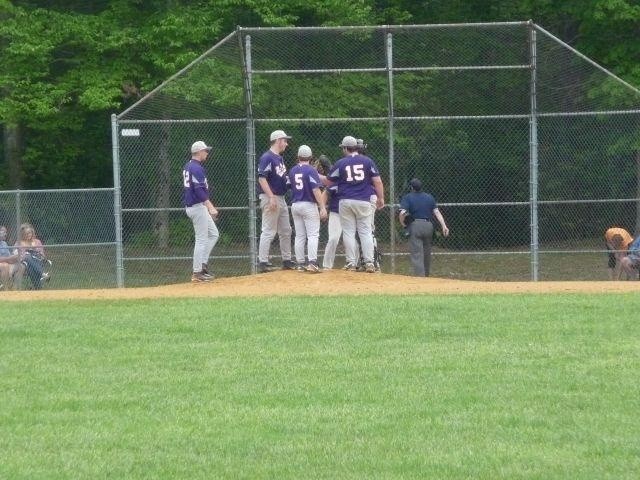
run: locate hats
[270,130,292,141]
[298,145,312,157]
[191,141,212,154]
[338,136,358,147]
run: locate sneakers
[260,262,280,274]
[192,263,217,284]
[283,261,323,273]
[341,261,376,273]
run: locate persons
[0,226,20,290]
[181,140,221,281]
[12,224,50,290]
[257,131,385,272]
[604,226,634,282]
[616,236,640,282]
[398,178,450,277]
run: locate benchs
[0,258,53,290]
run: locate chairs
[615,256,640,281]
